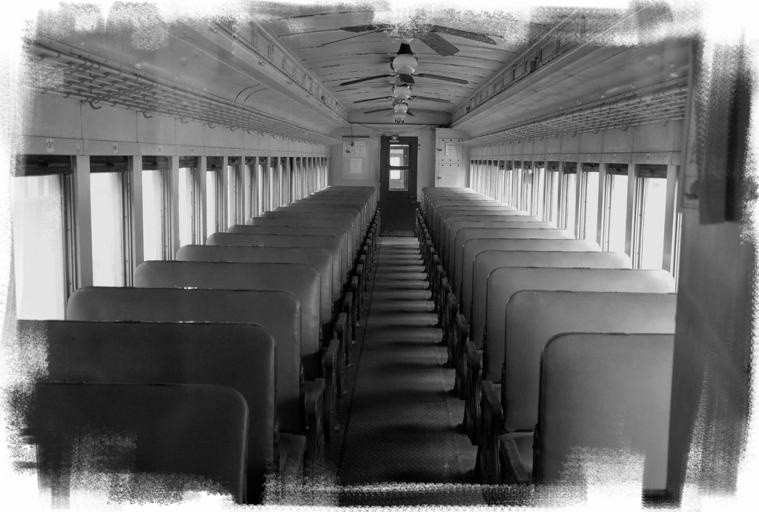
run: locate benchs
[453,238,675,503]
[10,186,380,341]
[13,236,351,501]
[416,186,677,371]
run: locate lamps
[392,85,413,100]
[394,114,406,122]
[391,99,409,114]
[392,42,418,75]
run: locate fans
[340,64,469,86]
[364,108,415,116]
[353,95,450,104]
[339,15,507,56]
